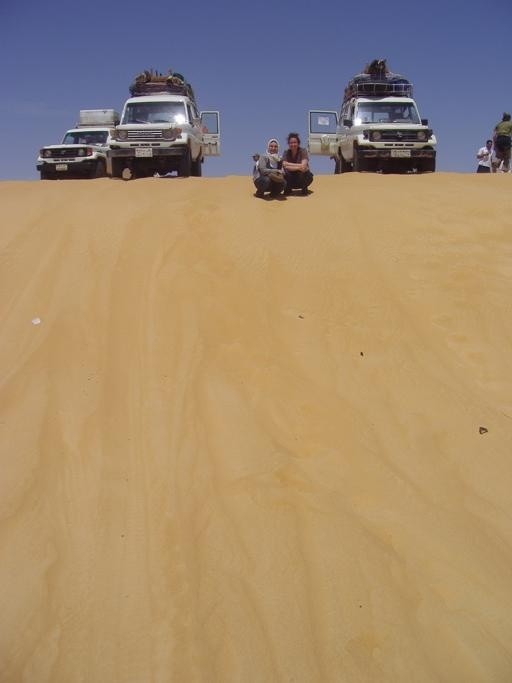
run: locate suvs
[307,56,440,175]
[38,107,121,180]
[109,68,226,181]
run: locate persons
[251,137,286,198]
[492,112,512,172]
[282,133,313,194]
[477,139,494,172]
